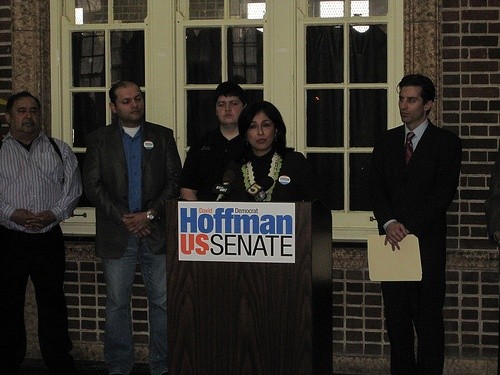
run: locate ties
[404,132,416,165]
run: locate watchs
[145,210,155,220]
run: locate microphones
[245,176,273,197]
[216,171,236,202]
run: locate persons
[485,149,500,251]
[372,74,462,375]
[217,100,319,203]
[179,81,248,201]
[0,91,88,375]
[82,82,183,375]
